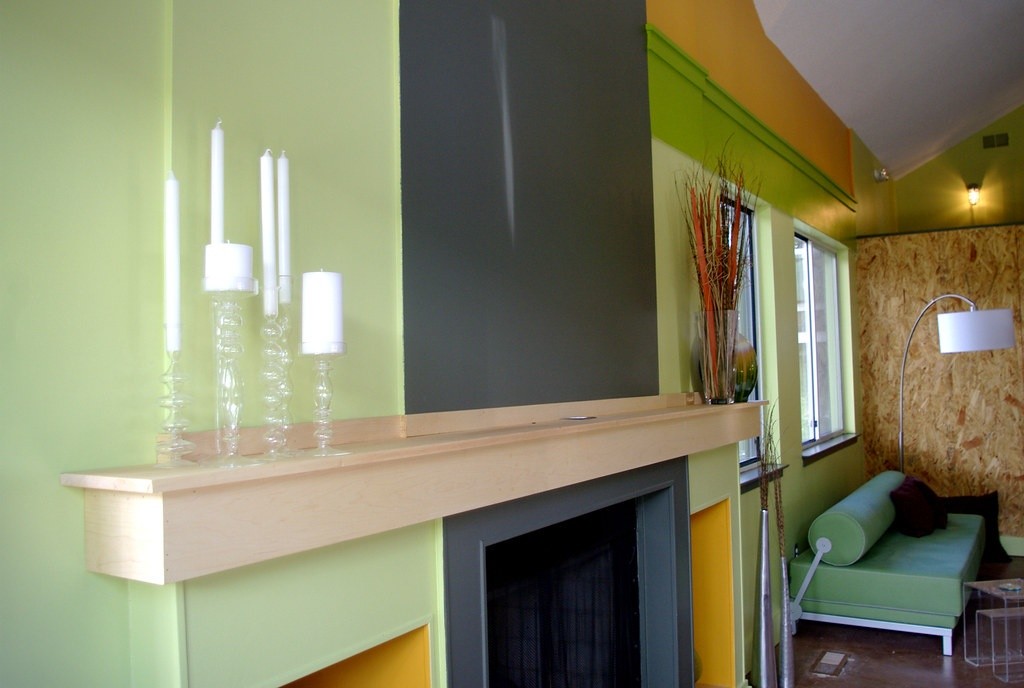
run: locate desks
[963,579,1024,684]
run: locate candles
[210,116,225,244]
[260,149,278,316]
[277,151,291,302]
[164,170,182,351]
[205,241,254,293]
[301,268,344,354]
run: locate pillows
[940,490,1012,562]
[889,476,948,535]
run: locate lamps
[875,168,889,180]
[899,295,1016,470]
[968,184,979,205]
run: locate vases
[698,310,739,405]
[698,333,758,403]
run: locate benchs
[787,470,986,656]
